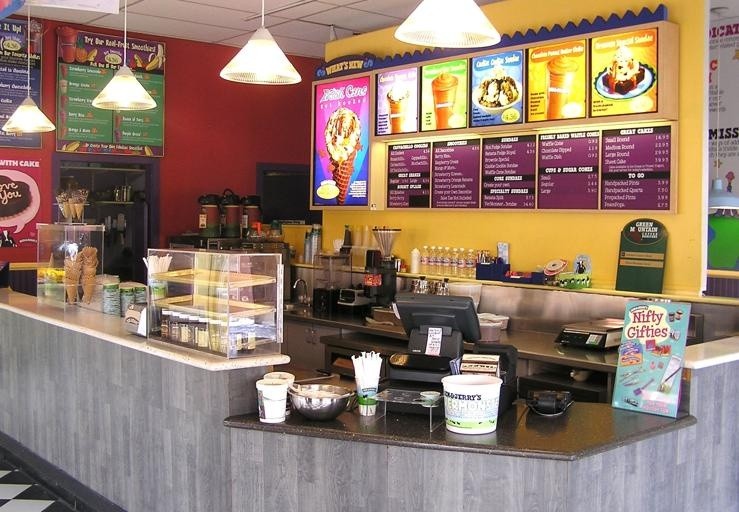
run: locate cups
[304,223,322,264]
[355,377,381,416]
[256,371,296,424]
[103,282,165,317]
[321,179,336,190]
[387,89,408,133]
[570,369,591,382]
[432,77,458,129]
[543,65,578,125]
[420,391,440,407]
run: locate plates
[600,67,652,101]
[317,186,340,199]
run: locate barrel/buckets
[256,380,287,423]
[263,371,296,389]
[441,375,504,435]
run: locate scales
[554,317,624,351]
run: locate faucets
[293,278,310,309]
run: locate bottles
[410,245,476,277]
[159,307,255,356]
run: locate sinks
[283,303,309,312]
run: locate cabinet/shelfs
[146,246,283,358]
[280,320,358,372]
[59,164,146,206]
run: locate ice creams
[323,106,362,205]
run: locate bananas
[145,44,166,72]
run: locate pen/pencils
[477,249,492,263]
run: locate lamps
[219,0,302,85]
[394,1,501,48]
[708,6,739,210]
[2,5,56,133]
[91,0,158,111]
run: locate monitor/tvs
[256,162,323,225]
[394,292,482,343]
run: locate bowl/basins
[472,83,523,114]
[287,384,355,421]
